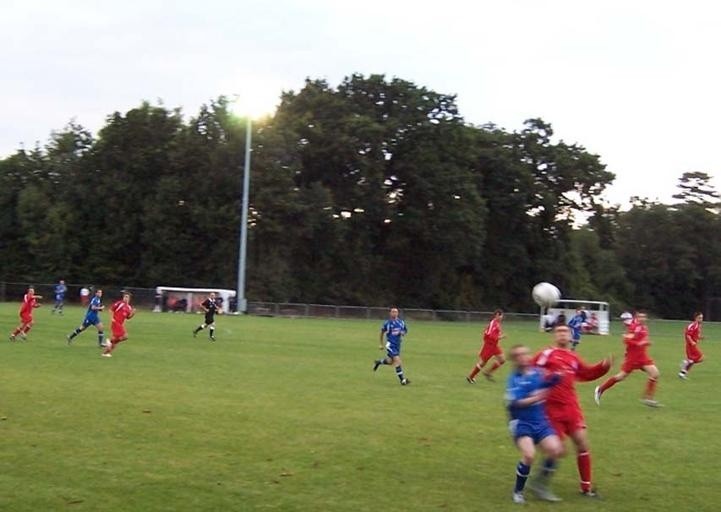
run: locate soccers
[532,282,561,308]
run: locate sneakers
[594,386,602,406]
[512,490,524,503]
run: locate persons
[620,309,633,327]
[373,307,411,386]
[529,306,616,499]
[191,293,219,340]
[594,309,660,408]
[8,285,43,341]
[51,280,107,348]
[466,308,508,384]
[678,311,708,382]
[503,344,564,505]
[166,295,187,313]
[101,291,137,357]
